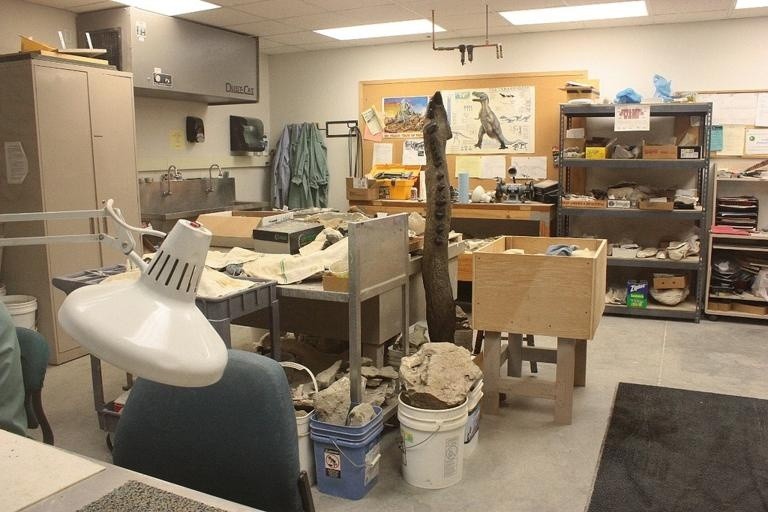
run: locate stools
[480,330,586,426]
[473,330,538,374]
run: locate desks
[350,197,558,240]
[0,427,270,512]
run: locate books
[715,197,760,232]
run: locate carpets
[585,382,767,512]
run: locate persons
[2,300,27,436]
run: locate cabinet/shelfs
[559,100,711,322]
[705,163,768,321]
[0,54,144,365]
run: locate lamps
[0,198,229,389]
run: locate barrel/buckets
[0,291,40,336]
[466,377,487,455]
[274,358,318,490]
[309,400,384,501]
[399,391,468,491]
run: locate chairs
[112,350,316,512]
[0,326,55,451]
[274,212,411,414]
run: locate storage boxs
[558,80,600,105]
[322,274,351,293]
[195,209,293,248]
[653,275,690,289]
[345,176,380,201]
[642,137,678,160]
[472,235,607,341]
